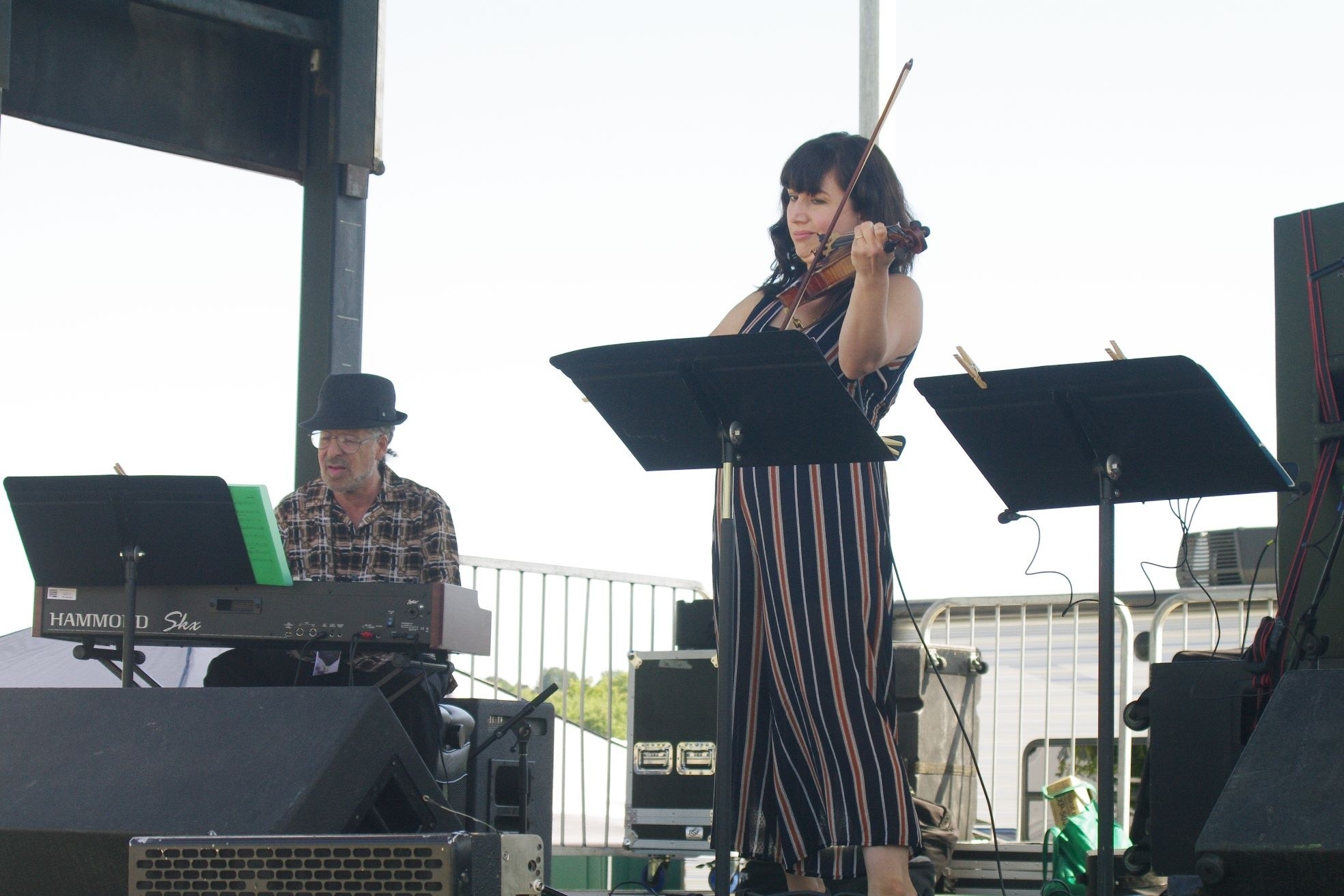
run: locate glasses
[307,429,385,455]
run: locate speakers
[0,685,553,896]
[1148,660,1344,896]
[1274,202,1344,668]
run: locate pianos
[29,581,495,688]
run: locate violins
[774,215,931,310]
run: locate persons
[707,130,930,896]
[204,383,462,832]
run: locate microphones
[997,513,1022,524]
[1290,481,1312,503]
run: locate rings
[856,236,864,241]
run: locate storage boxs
[1045,776,1091,828]
[623,650,718,851]
[1086,848,1168,891]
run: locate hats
[296,374,409,430]
